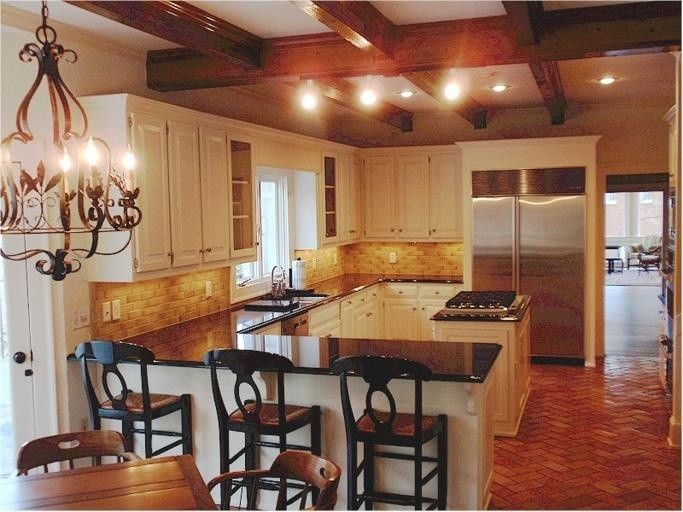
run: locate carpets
[605,264,661,285]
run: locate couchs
[627,243,644,269]
[637,245,660,271]
[605,245,628,268]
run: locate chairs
[15,431,142,475]
[74,338,194,466]
[334,355,448,511]
[206,451,341,511]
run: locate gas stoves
[430,291,533,321]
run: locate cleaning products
[292,257,305,290]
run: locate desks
[0,456,217,510]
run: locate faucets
[271,264,285,298]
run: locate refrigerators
[471,192,585,359]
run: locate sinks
[292,297,324,303]
[244,300,304,310]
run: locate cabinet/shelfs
[205,348,320,511]
[70,93,204,283]
[459,138,605,368]
[199,109,258,271]
[363,145,466,242]
[431,295,532,437]
[306,271,385,340]
[385,275,464,339]
[292,134,361,252]
[659,104,678,398]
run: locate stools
[605,257,621,273]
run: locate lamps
[0,0,144,281]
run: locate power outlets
[111,300,123,319]
